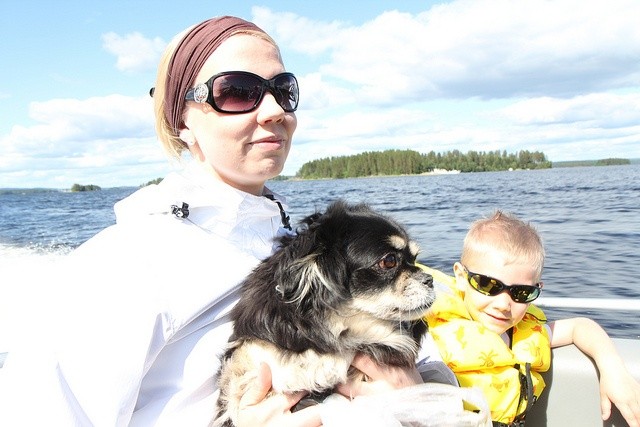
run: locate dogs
[217,199,436,427]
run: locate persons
[416,212,640,427]
[3,15,492,427]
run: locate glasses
[460,263,542,303]
[151,71,299,113]
